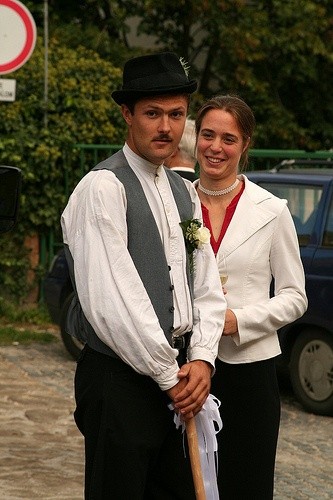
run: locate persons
[61,52,228,500]
[189,95,308,500]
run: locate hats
[111,53,197,106]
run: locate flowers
[181,220,210,256]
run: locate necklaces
[198,178,241,196]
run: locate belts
[172,333,191,348]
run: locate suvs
[42,158,333,418]
